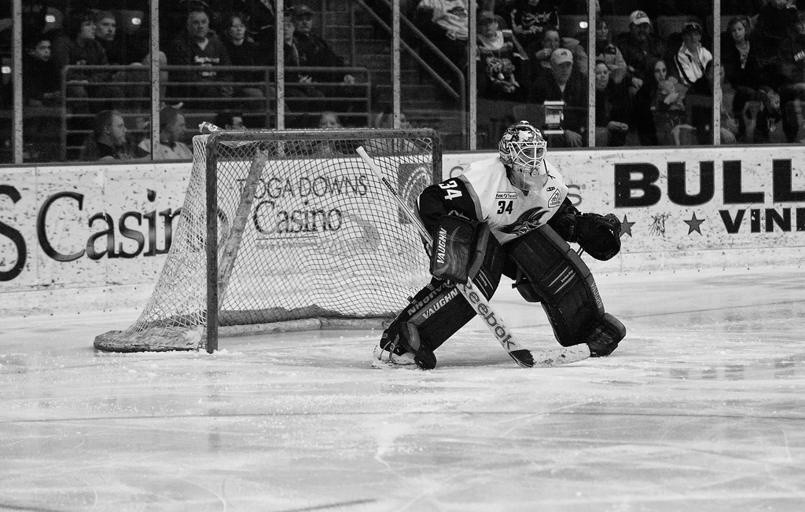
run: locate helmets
[499,120,548,192]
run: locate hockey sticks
[355,146,592,369]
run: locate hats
[627,10,652,28]
[551,48,574,65]
[293,4,316,17]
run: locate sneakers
[373,343,416,365]
[557,343,591,364]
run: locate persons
[477,1,805,147]
[371,120,626,369]
[417,0,469,54]
[1,1,352,162]
[368,113,418,155]
